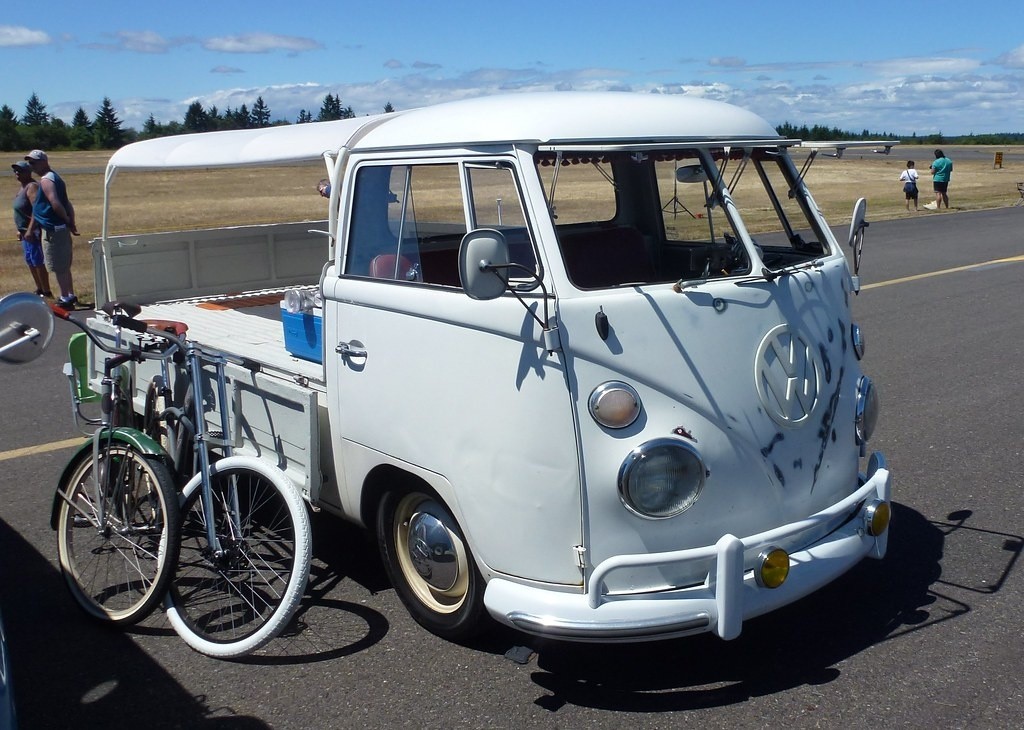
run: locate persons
[930,149,953,209]
[24,149,81,312]
[11,161,56,300]
[899,160,920,212]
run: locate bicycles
[46,300,313,659]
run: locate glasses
[319,186,328,193]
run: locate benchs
[1015,182,1024,206]
[402,228,658,288]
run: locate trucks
[88,92,891,645]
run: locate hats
[12,162,30,169]
[25,149,49,161]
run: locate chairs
[370,252,415,282]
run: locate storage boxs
[280,299,322,364]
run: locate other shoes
[71,295,79,306]
[34,289,42,295]
[39,291,53,300]
[54,298,75,310]
